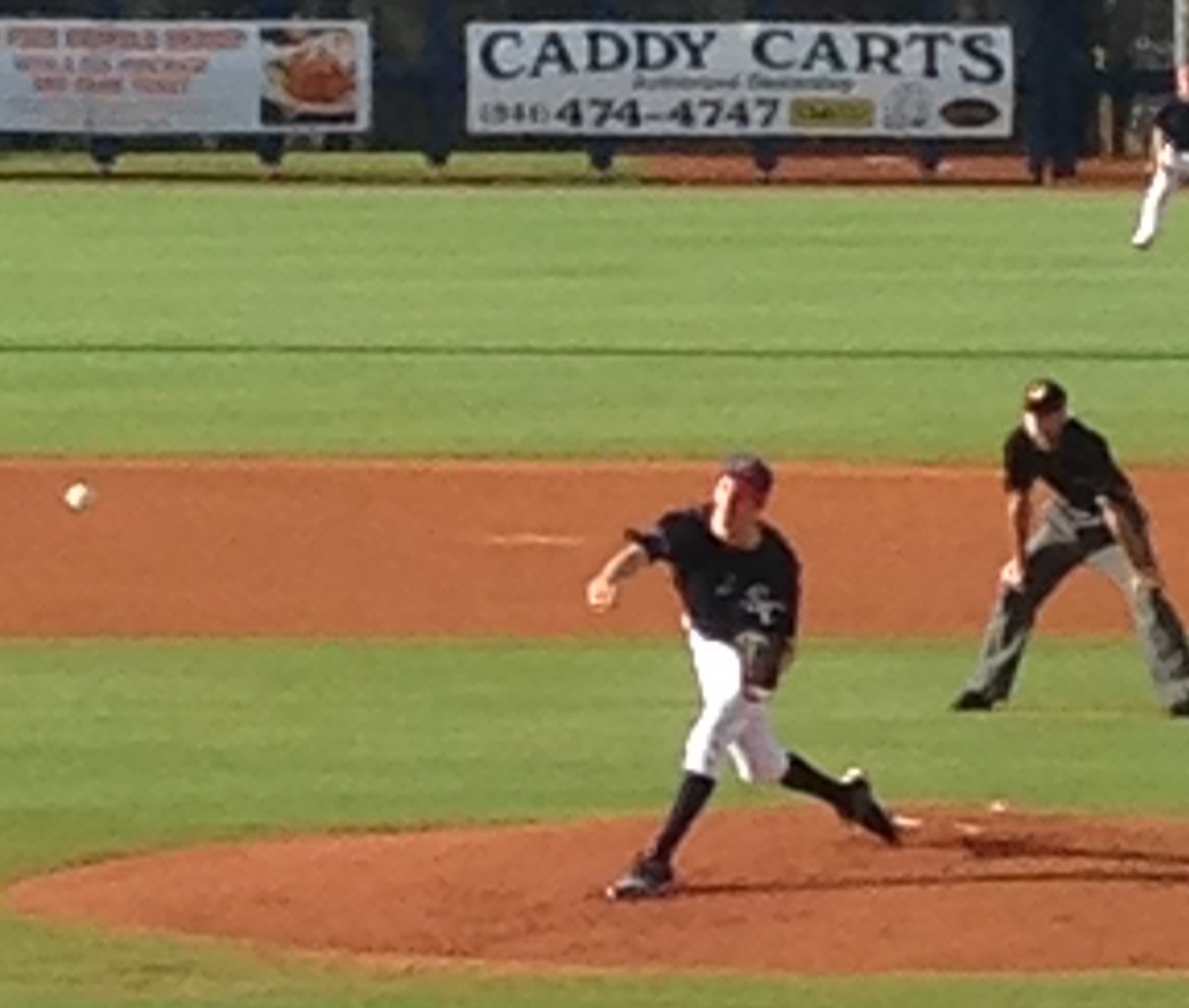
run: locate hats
[722,455,772,496]
[1022,380,1064,414]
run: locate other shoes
[1171,700,1189,716]
[955,689,988,710]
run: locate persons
[585,454,904,904]
[947,381,1189,719]
[1131,63,1189,250]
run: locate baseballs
[64,482,88,509]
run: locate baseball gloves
[734,626,789,688]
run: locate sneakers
[842,766,897,845]
[604,852,673,902]
